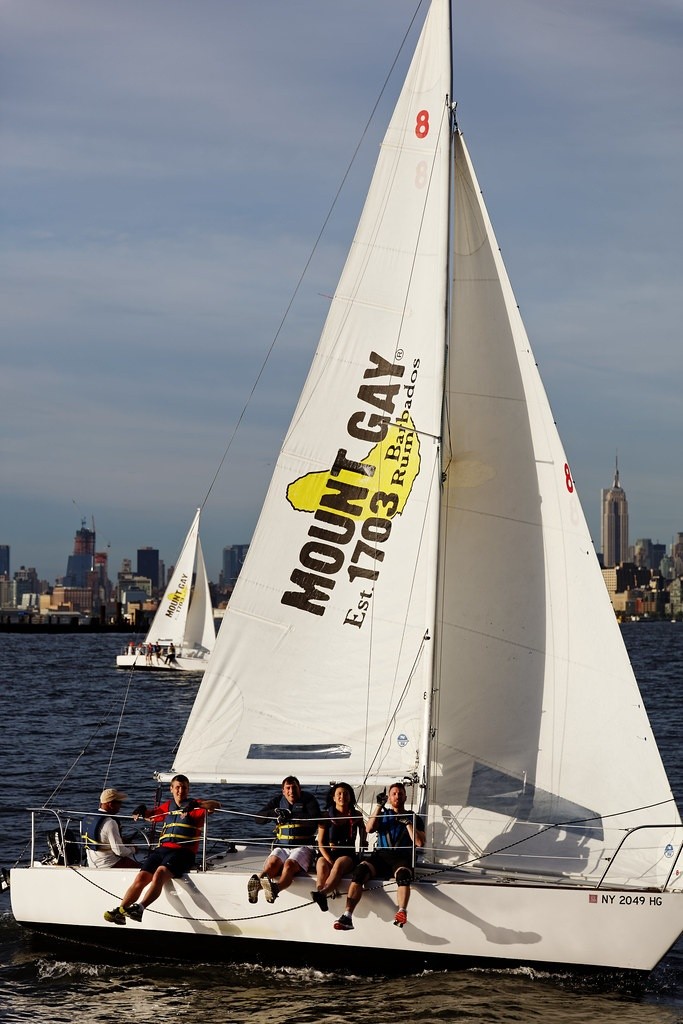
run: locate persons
[82,790,143,868]
[126,642,175,667]
[334,783,426,930]
[248,775,321,904]
[310,783,367,910]
[104,775,220,925]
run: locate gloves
[376,787,388,806]
[275,808,293,825]
[395,813,412,826]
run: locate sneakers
[103,907,126,925]
[393,909,407,928]
[119,903,143,922]
[333,915,354,930]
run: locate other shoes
[310,891,328,911]
[260,873,279,903]
[247,874,260,903]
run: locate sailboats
[0,0,682,971]
[116,507,216,671]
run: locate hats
[100,789,128,804]
